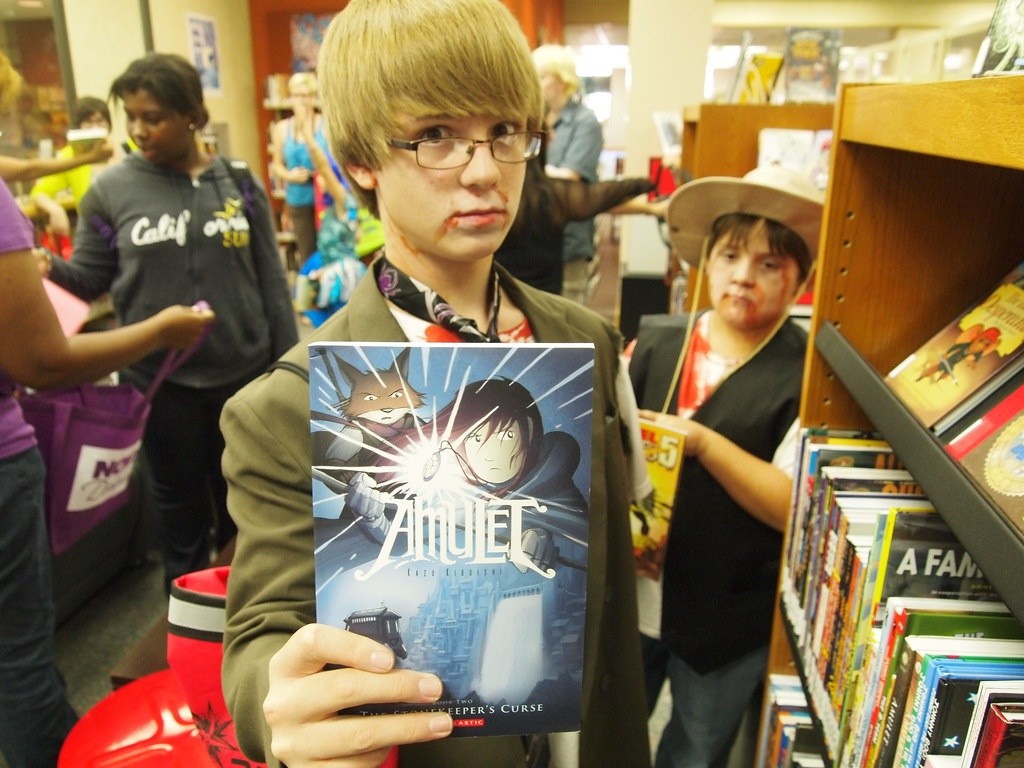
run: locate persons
[215,0,656,768]
[293,110,385,263]
[1,175,210,768]
[497,110,657,299]
[273,67,332,266]
[0,55,112,180]
[37,51,301,599]
[625,166,825,768]
[529,52,603,306]
[35,96,135,210]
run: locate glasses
[386,128,548,170]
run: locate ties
[373,257,502,345]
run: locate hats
[664,165,824,271]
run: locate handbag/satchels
[18,301,212,559]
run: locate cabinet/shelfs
[665,72,1024,764]
